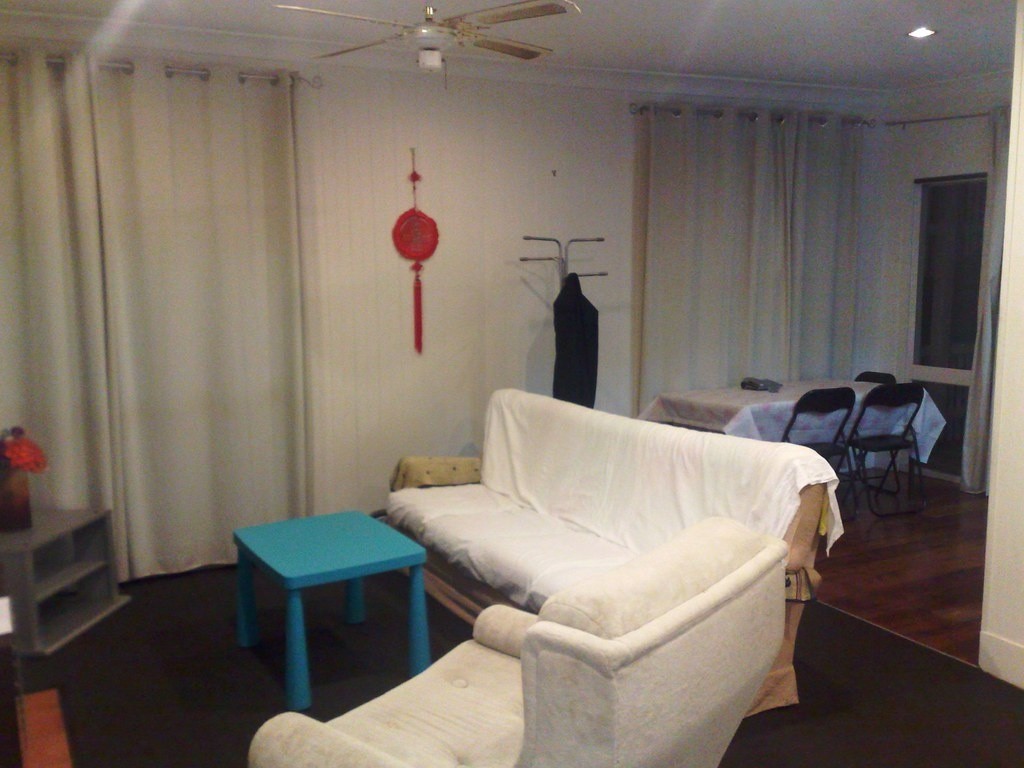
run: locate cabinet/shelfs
[0,506,133,661]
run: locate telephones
[741,377,783,391]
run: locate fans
[269,1,581,62]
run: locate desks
[639,379,946,484]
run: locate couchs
[387,388,843,717]
[248,517,789,768]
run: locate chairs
[779,388,855,517]
[846,371,926,518]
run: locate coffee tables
[230,510,430,714]
